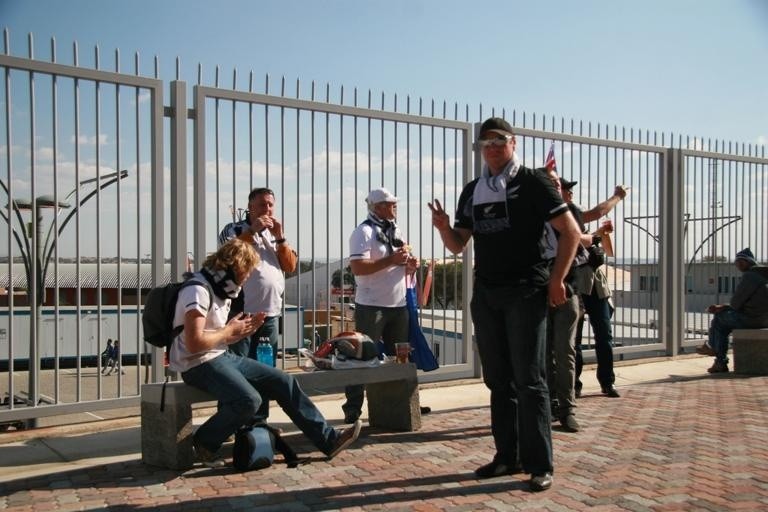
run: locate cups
[602,219,613,234]
[394,341,410,364]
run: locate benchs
[141,359,423,470]
[732,328,768,374]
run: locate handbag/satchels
[297,328,380,367]
[234,421,311,472]
[405,268,439,371]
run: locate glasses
[478,136,513,146]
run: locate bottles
[262,336,274,368]
[256,336,266,362]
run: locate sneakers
[193,434,226,468]
[327,411,362,458]
[696,340,728,373]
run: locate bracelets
[270,238,285,244]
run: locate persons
[104,341,125,376]
[696,247,768,373]
[217,187,299,442]
[164,237,362,472]
[430,118,582,490]
[340,188,439,425]
[537,168,628,433]
[100,339,114,375]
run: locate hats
[480,118,512,136]
[736,248,757,266]
[366,188,398,204]
[559,177,577,189]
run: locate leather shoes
[475,461,553,490]
[560,414,580,432]
[601,382,620,397]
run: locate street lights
[2,195,69,406]
[229,206,249,222]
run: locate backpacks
[142,272,212,412]
